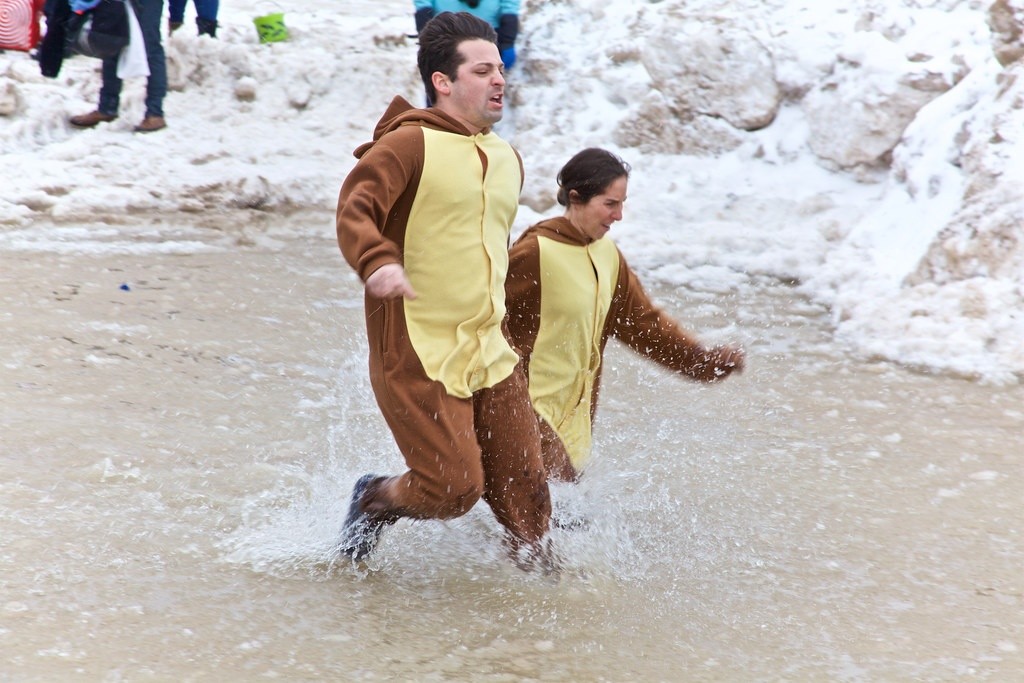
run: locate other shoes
[337,473,396,566]
[73,112,118,126]
[135,115,168,132]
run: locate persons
[30,0,222,135]
[338,12,560,579]
[412,0,519,108]
[504,146,750,531]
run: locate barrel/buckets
[252,0,288,44]
